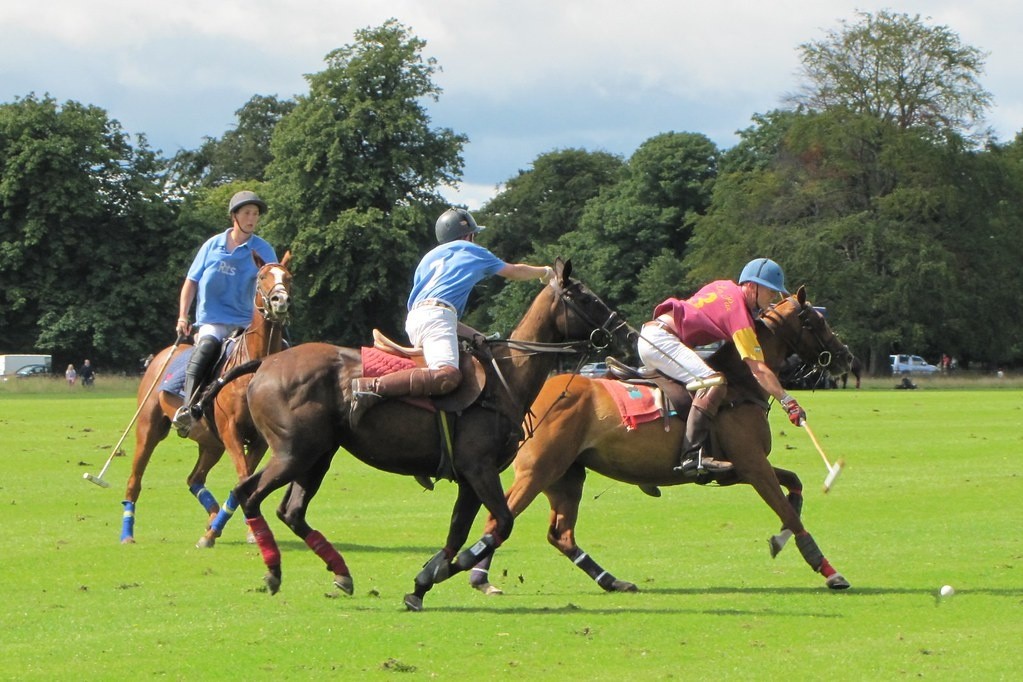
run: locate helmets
[228,191,266,218]
[736,258,789,295]
[435,205,485,243]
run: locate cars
[578,361,639,380]
[2,365,54,384]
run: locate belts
[643,321,678,337]
[411,300,455,312]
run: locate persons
[348,207,557,428]
[79,359,95,390]
[939,353,958,375]
[65,364,76,392]
[172,190,291,438]
[637,258,807,476]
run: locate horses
[467,283,854,592]
[192,254,638,612]
[841,355,862,389]
[120,248,291,544]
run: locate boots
[175,336,220,438]
[349,366,462,430]
[680,372,733,477]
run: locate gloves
[782,396,806,427]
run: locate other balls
[941,585,954,596]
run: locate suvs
[889,354,940,378]
